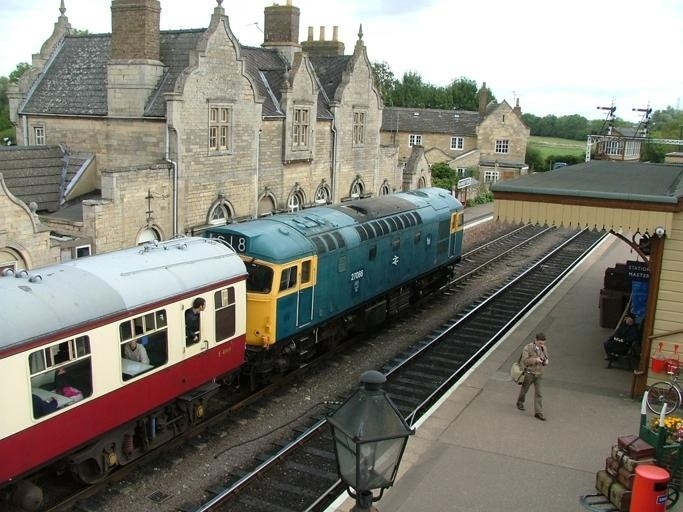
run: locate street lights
[324,370,415,512]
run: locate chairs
[606,338,641,370]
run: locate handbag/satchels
[510,363,525,385]
[608,336,630,355]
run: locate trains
[0,187,467,512]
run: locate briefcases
[596,435,656,510]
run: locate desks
[122,356,155,378]
[32,387,74,410]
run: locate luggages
[599,263,632,328]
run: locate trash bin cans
[629,465,672,512]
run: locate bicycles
[646,368,683,416]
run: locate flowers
[649,415,683,443]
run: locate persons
[125,336,150,365]
[517,333,549,421]
[186,297,206,342]
[45,374,85,407]
[603,312,640,360]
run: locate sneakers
[517,402,526,410]
[535,413,545,420]
